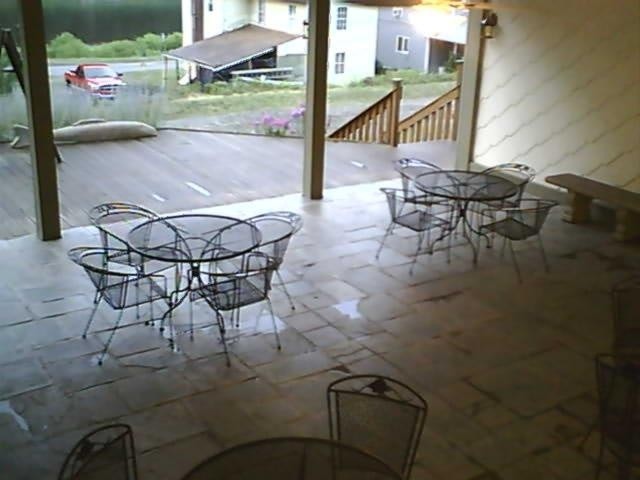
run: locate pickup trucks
[63,64,127,102]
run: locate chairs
[58,423,137,480]
[327,375,428,480]
[87,202,180,307]
[374,188,454,276]
[593,352,640,480]
[464,164,537,248]
[202,211,302,328]
[67,247,175,366]
[612,276,640,363]
[473,196,559,285]
[392,157,458,241]
[188,254,284,369]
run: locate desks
[412,170,518,265]
[176,438,404,480]
[126,213,262,342]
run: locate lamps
[481,12,498,39]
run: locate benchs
[546,174,640,242]
